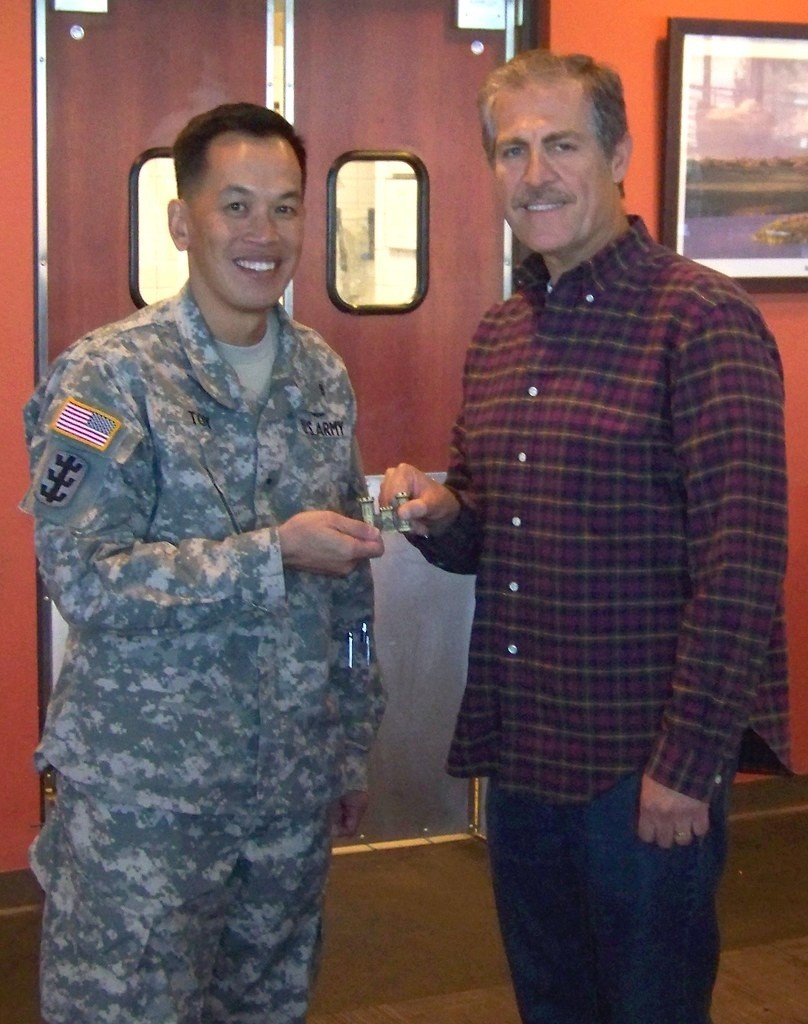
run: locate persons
[378,47,793,1024]
[24,100,384,1024]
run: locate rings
[674,831,692,837]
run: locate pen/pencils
[362,622,370,666]
[347,631,353,668]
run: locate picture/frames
[655,17,808,293]
